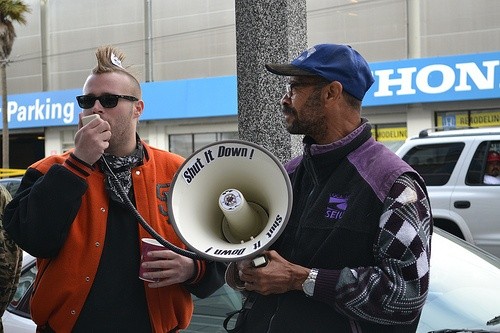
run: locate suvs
[380,125,500,257]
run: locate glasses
[76,93,138,108]
[287,80,330,93]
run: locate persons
[225,44,434,333]
[0,46,230,333]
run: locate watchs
[301,268,320,298]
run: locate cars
[0,176,23,197]
[1,224,500,333]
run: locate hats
[264,44,375,101]
[487,151,500,161]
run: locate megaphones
[167,139,294,262]
